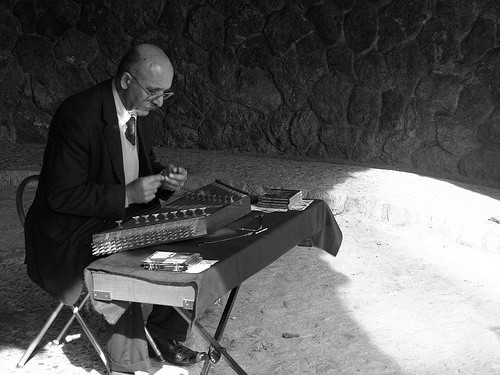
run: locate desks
[89,198,328,375]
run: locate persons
[24,44,207,375]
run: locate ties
[125,116,136,147]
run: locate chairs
[17,174,167,375]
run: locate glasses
[134,78,175,102]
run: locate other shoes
[148,339,205,366]
